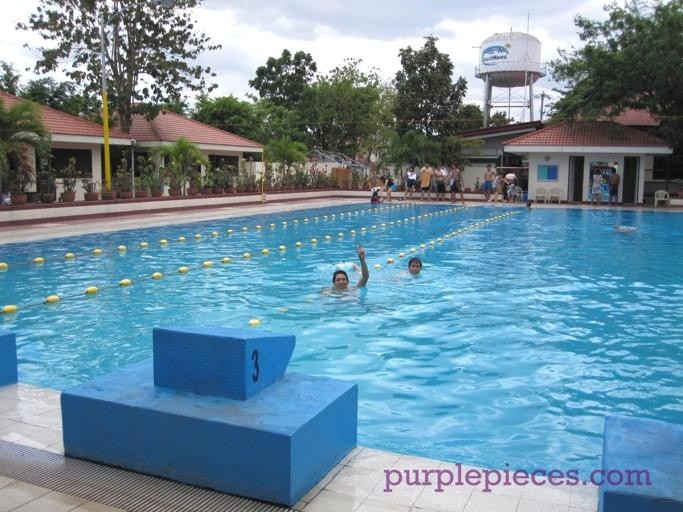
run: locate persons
[321,243,369,295]
[608,167,620,206]
[408,257,423,275]
[591,168,602,206]
[526,200,532,207]
[370,162,519,206]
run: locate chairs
[512,186,523,202]
[548,188,561,205]
[534,188,546,205]
[654,190,669,208]
